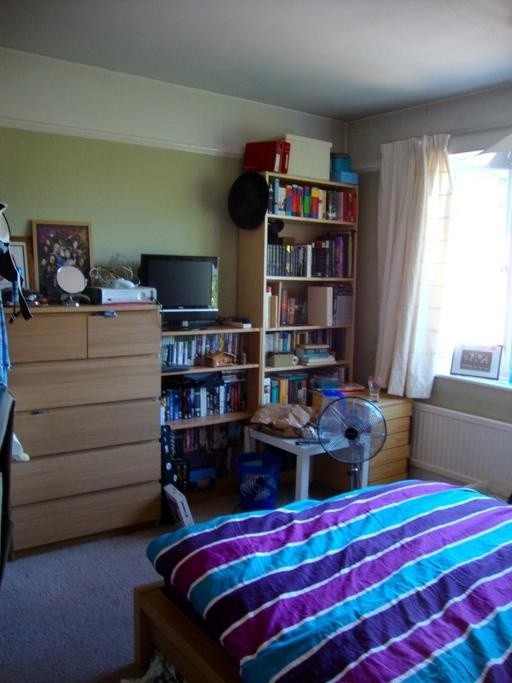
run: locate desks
[244,425,371,500]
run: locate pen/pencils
[296,440,330,445]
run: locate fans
[317,397,387,490]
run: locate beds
[132,480,511,683]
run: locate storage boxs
[267,134,333,182]
[331,172,359,184]
[331,157,352,171]
[244,140,290,172]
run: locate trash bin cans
[233,452,283,511]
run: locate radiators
[412,401,512,499]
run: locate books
[164,483,195,528]
[260,176,358,408]
[159,333,250,456]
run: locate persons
[41,233,88,297]
[462,351,490,369]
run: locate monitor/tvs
[140,253,221,331]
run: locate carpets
[1,528,177,683]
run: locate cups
[368,376,381,401]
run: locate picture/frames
[31,219,94,305]
[10,236,35,292]
[450,343,503,379]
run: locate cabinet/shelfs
[161,325,262,493]
[238,172,359,410]
[311,389,412,490]
[0,305,161,561]
